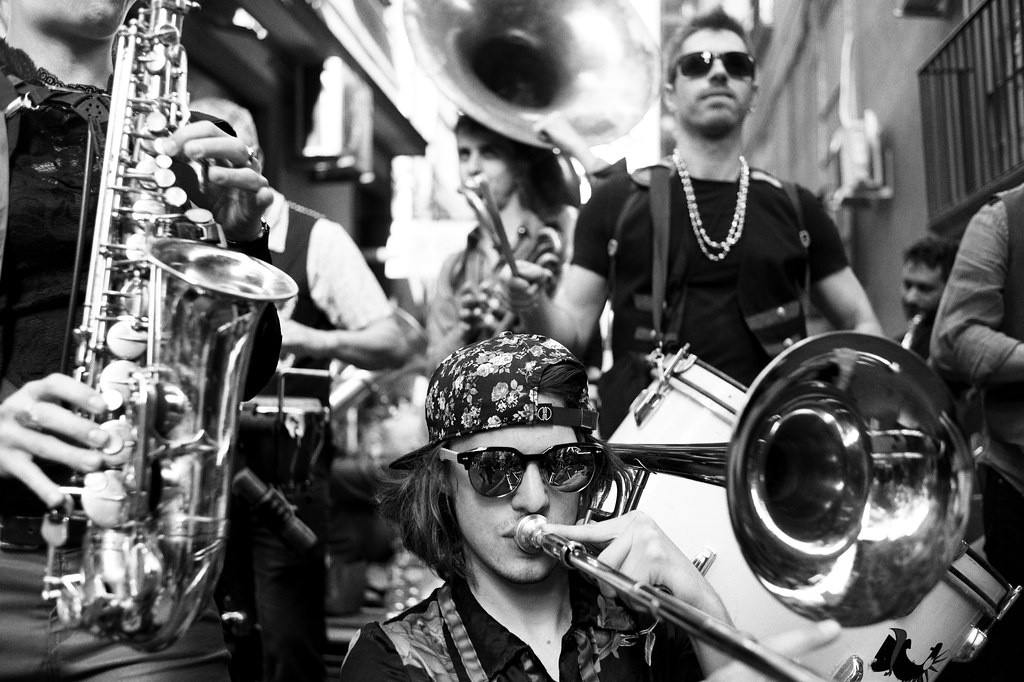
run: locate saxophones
[38,0,304,656]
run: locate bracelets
[226,217,268,243]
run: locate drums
[575,343,1017,682]
[236,394,330,549]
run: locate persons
[412,115,604,386]
[891,236,961,362]
[495,4,884,444]
[927,180,1024,682]
[187,99,408,682]
[0,0,270,682]
[337,332,842,682]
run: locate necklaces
[672,148,750,261]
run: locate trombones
[512,328,981,681]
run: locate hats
[389,331,600,472]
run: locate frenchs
[399,0,665,343]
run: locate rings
[246,146,254,164]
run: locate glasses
[437,442,604,499]
[669,52,756,83]
